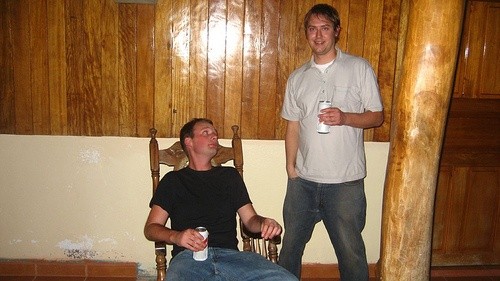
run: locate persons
[277,2,384,280]
[144,118,298,280]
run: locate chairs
[148,124,282,281]
